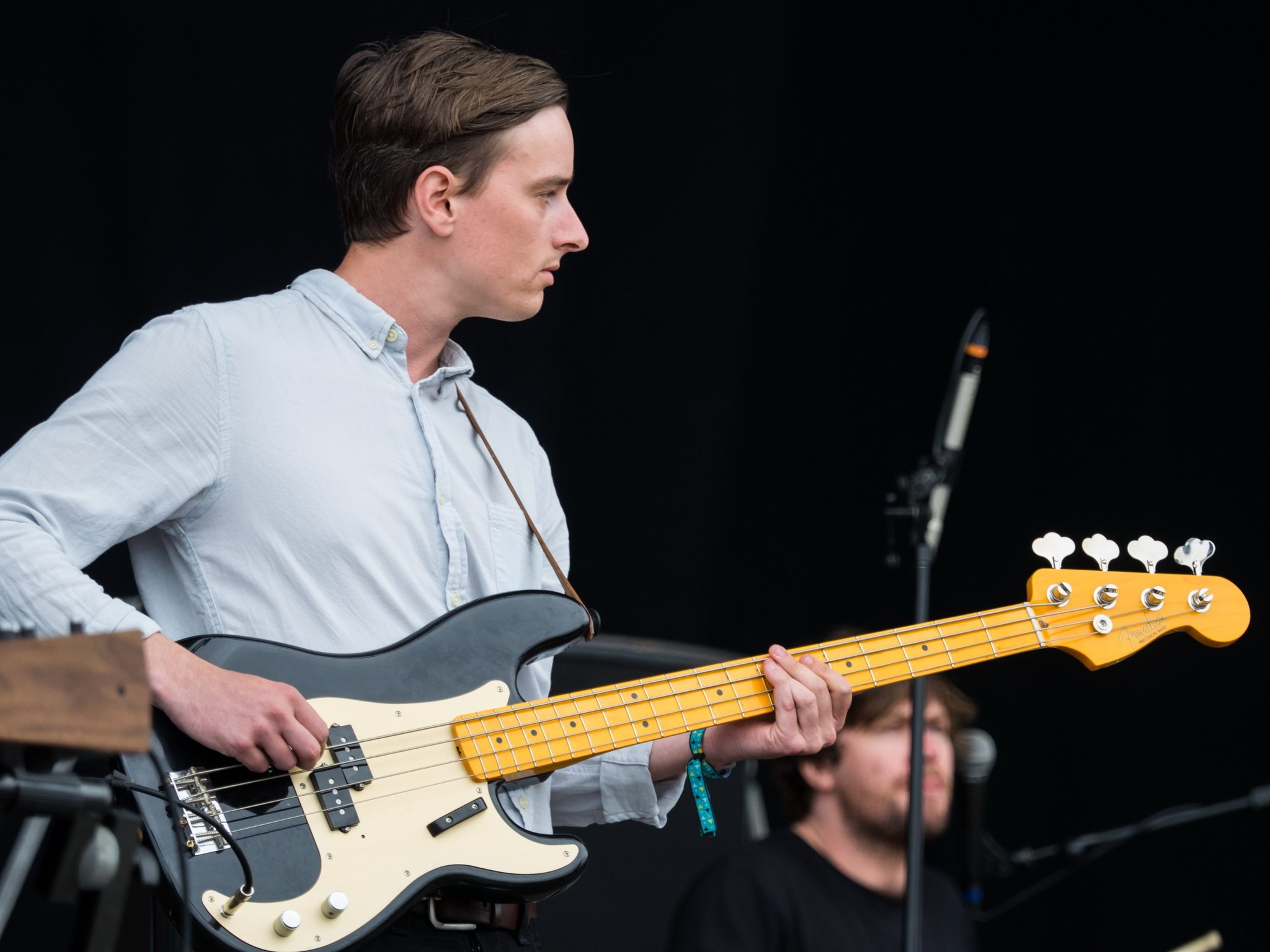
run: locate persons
[658,625,984,952]
[0,32,851,951]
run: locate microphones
[920,326,993,547]
[955,731,994,908]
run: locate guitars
[116,529,1253,952]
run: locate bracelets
[685,728,734,838]
